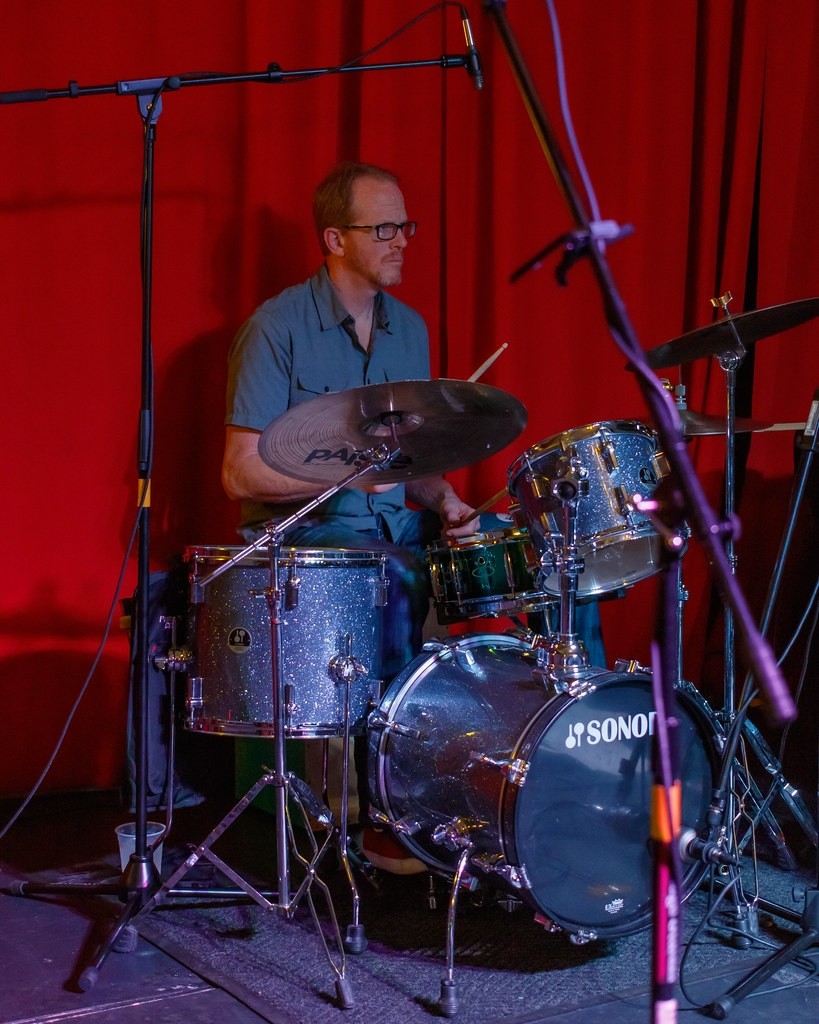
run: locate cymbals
[258,381,529,484]
[643,411,808,436]
[624,296,819,372]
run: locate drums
[188,547,387,739]
[508,417,689,596]
[369,634,730,945]
[425,526,563,627]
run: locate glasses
[344,221,417,241]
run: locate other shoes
[350,824,428,875]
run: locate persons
[222,159,483,874]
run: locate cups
[114,821,167,876]
